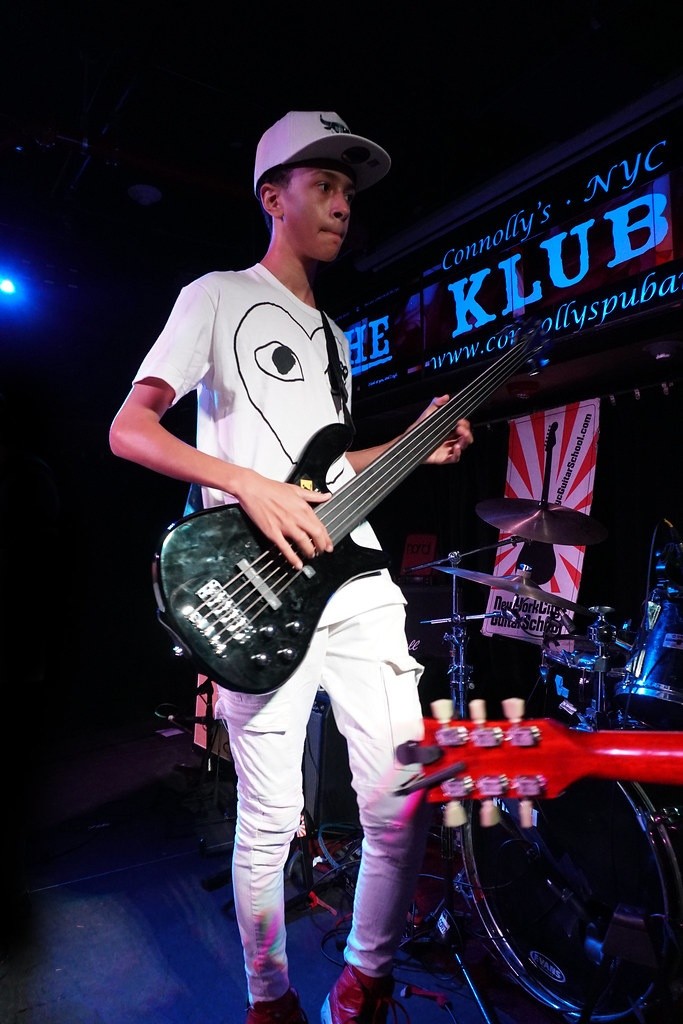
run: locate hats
[253,110,392,201]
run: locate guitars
[391,699,682,831]
[146,320,566,696]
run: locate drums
[612,578,682,728]
[538,631,634,729]
[428,776,682,1024]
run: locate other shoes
[245,986,309,1024]
[320,964,412,1024]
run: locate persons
[107,108,474,1024]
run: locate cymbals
[430,562,602,619]
[474,495,617,546]
